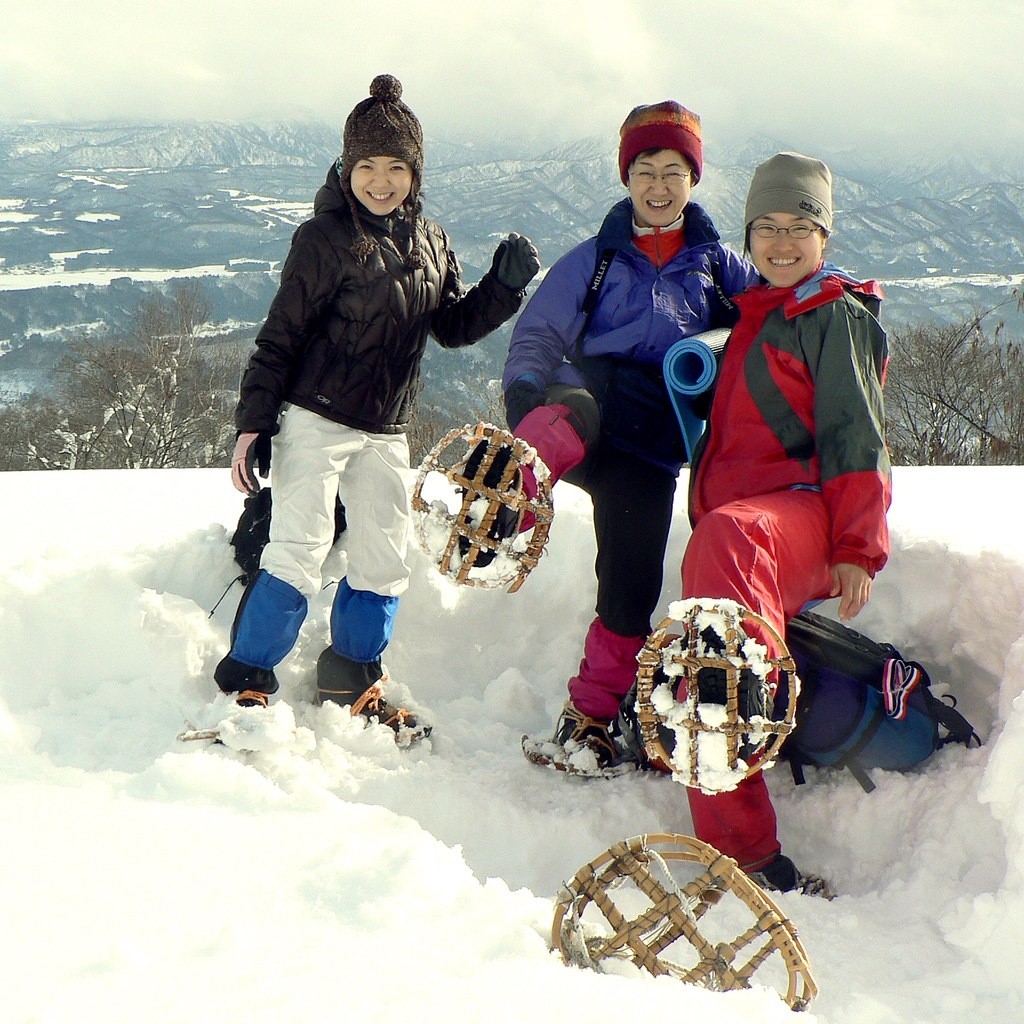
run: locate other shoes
[749,854,802,894]
[225,693,271,710]
[561,715,614,767]
[458,442,518,567]
[312,648,434,739]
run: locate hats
[339,74,427,270]
[618,99,703,188]
[745,152,833,233]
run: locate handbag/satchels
[774,611,981,794]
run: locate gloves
[491,231,542,292]
[230,432,271,500]
[504,376,545,431]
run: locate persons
[212,74,540,750]
[675,151,893,891]
[458,100,759,769]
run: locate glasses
[749,223,821,240]
[626,168,693,186]
